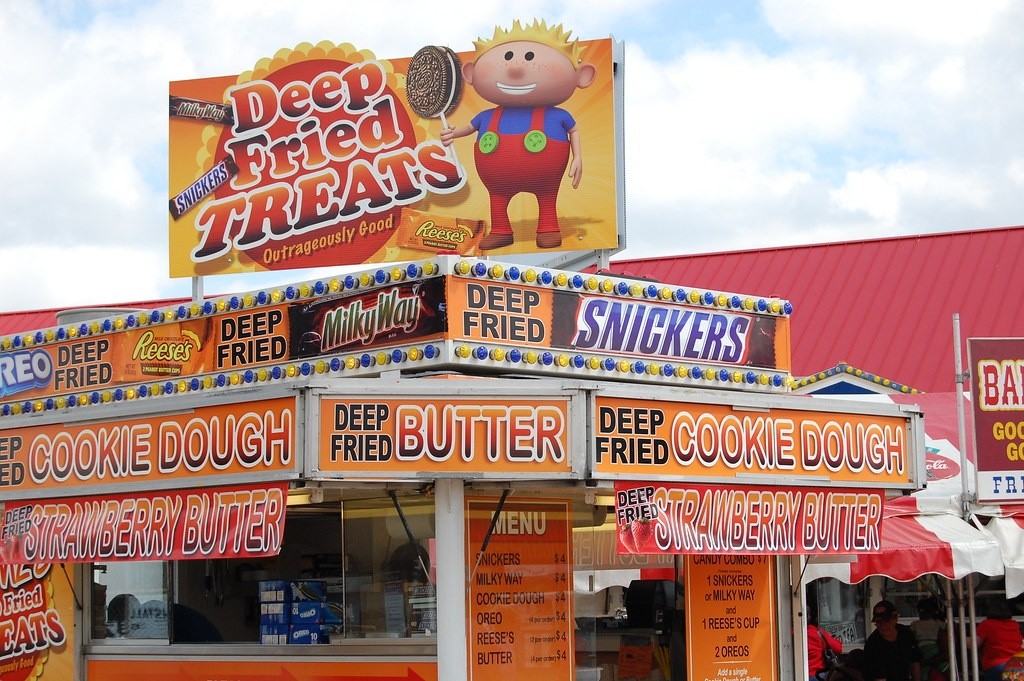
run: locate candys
[169,95,234,124]
[169,154,240,222]
[398,207,486,257]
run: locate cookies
[406,44,464,118]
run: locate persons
[910,600,970,680]
[864,601,923,681]
[807,600,842,681]
[966,599,1022,681]
[830,649,865,681]
[106,593,223,644]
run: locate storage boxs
[260,603,289,625]
[289,624,361,644]
[258,578,327,604]
[290,602,361,624]
[259,624,289,644]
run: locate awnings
[971,500,1024,600]
[793,497,1004,594]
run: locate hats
[871,601,896,622]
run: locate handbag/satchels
[822,646,839,667]
